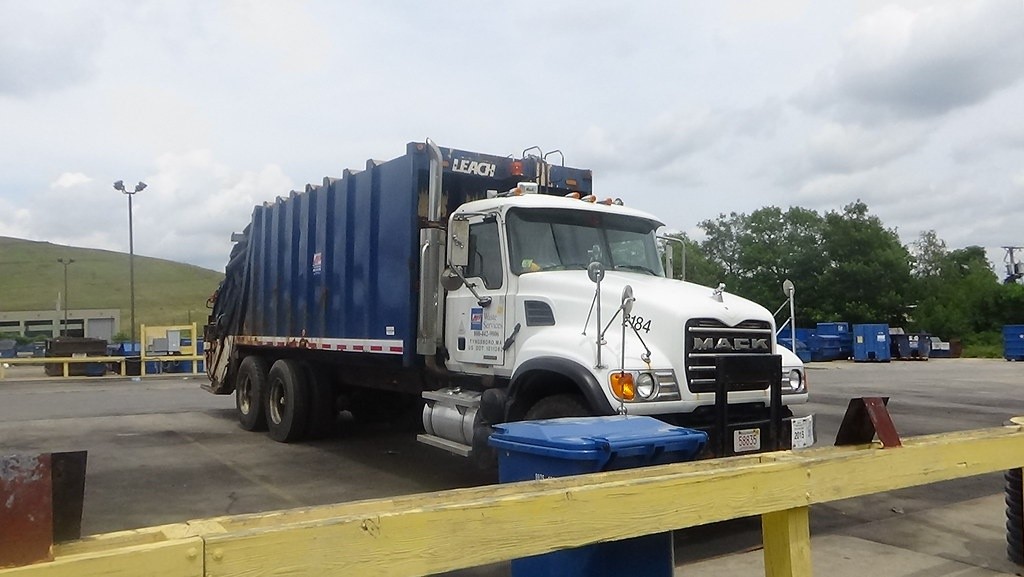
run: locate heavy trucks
[203,140,808,473]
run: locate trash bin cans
[909,336,951,357]
[486,415,711,577]
[44,335,108,377]
[889,333,931,361]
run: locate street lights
[56,256,75,337]
[113,180,148,350]
